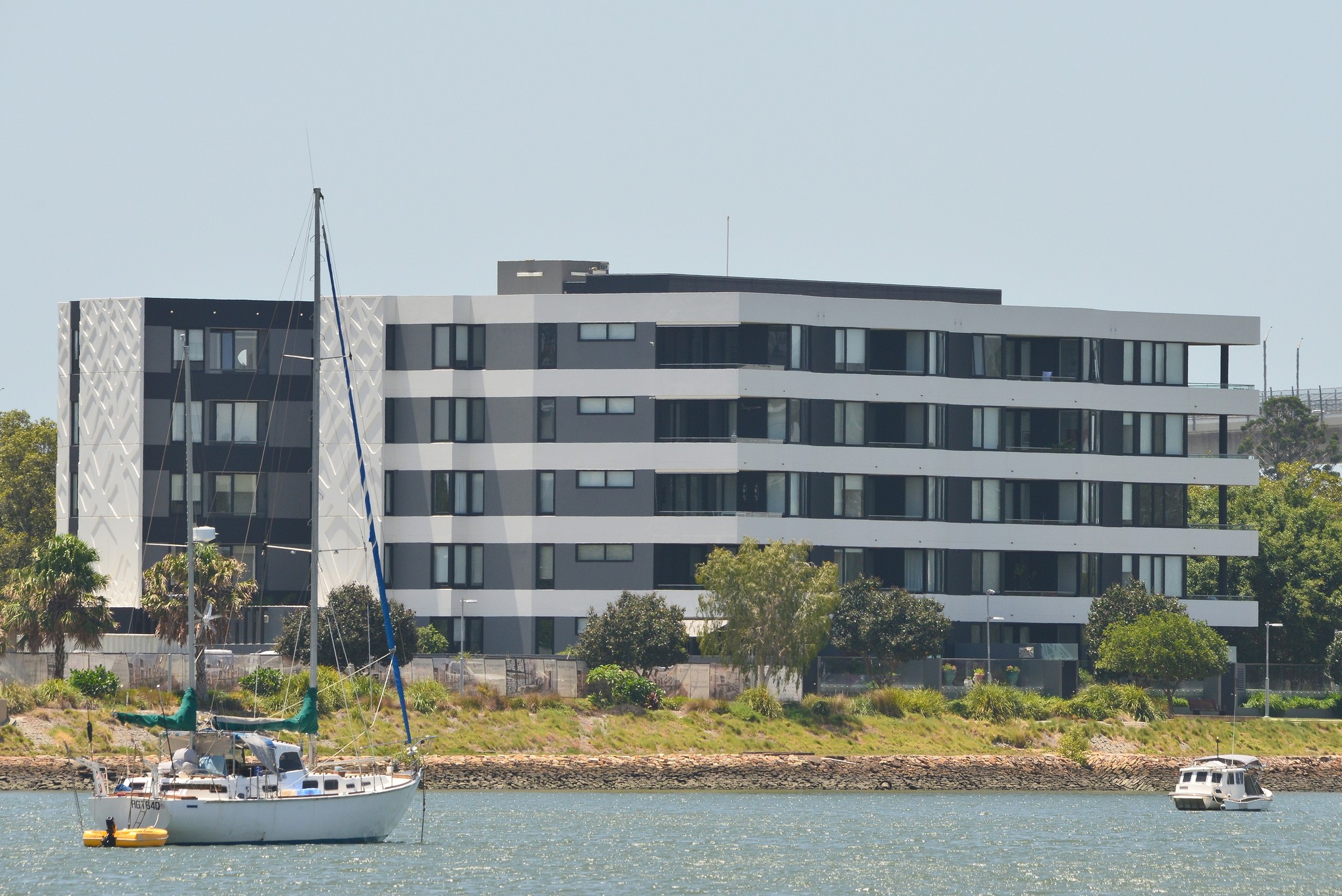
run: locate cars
[446,661,514,694]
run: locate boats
[83,826,169,848]
[1166,692,1274,811]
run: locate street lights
[459,597,477,697]
[1264,622,1284,719]
[987,615,1005,685]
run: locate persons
[279,753,289,773]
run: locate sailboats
[76,184,429,844]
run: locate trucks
[202,649,235,692]
